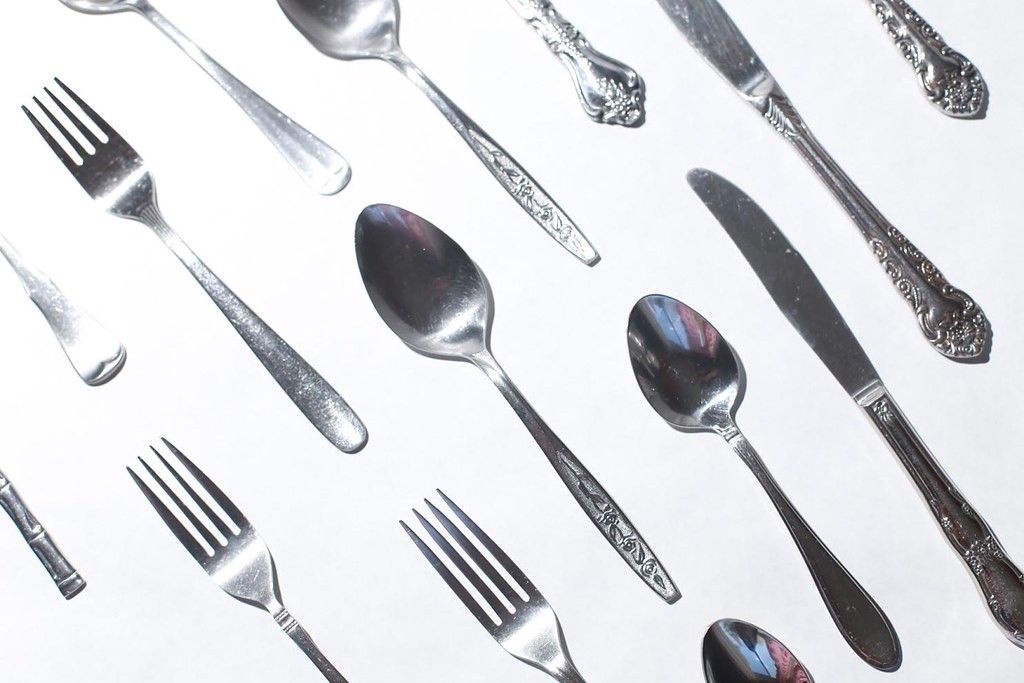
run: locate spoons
[275,0,601,266]
[354,202,680,604]
[57,0,351,197]
[626,294,904,674]
[702,617,811,682]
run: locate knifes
[656,0,991,360]
[684,169,1023,648]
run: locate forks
[398,486,586,683]
[125,438,349,682]
[19,77,368,456]
